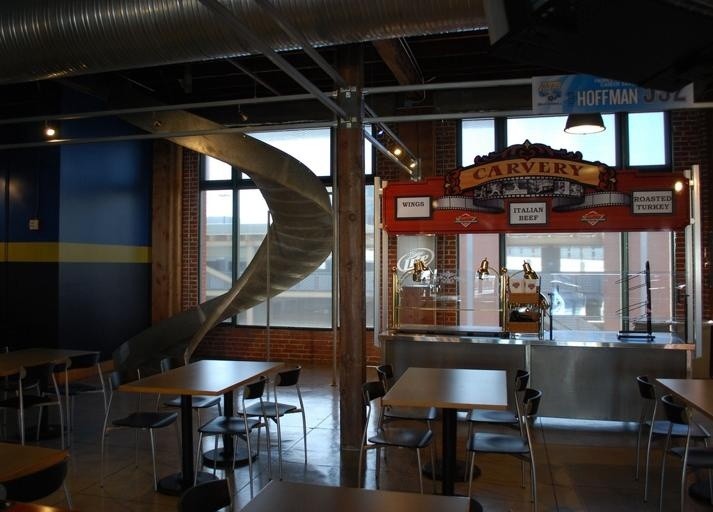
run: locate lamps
[478,258,549,332]
[373,124,421,181]
[392,261,433,330]
[565,113,606,134]
